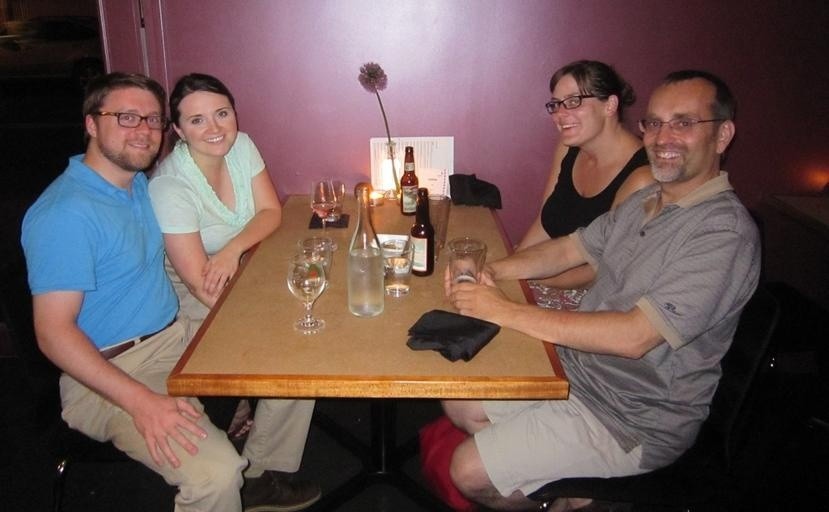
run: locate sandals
[227,418,253,441]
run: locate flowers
[358,61,402,193]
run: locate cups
[448,238,487,295]
[304,237,333,290]
[383,240,414,297]
[327,182,345,222]
[428,194,451,248]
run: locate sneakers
[241,468,323,512]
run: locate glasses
[638,117,724,134]
[545,93,607,115]
[98,110,168,130]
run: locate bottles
[401,146,419,216]
[348,184,384,317]
[410,188,435,276]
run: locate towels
[449,174,502,209]
[406,310,501,362]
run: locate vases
[381,142,402,200]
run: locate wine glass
[286,254,325,334]
[311,179,337,251]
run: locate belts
[98,316,177,361]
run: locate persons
[514,61,657,310]
[441,71,763,511]
[20,73,322,512]
[146,74,282,438]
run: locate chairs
[530,283,783,512]
[0,265,259,512]
